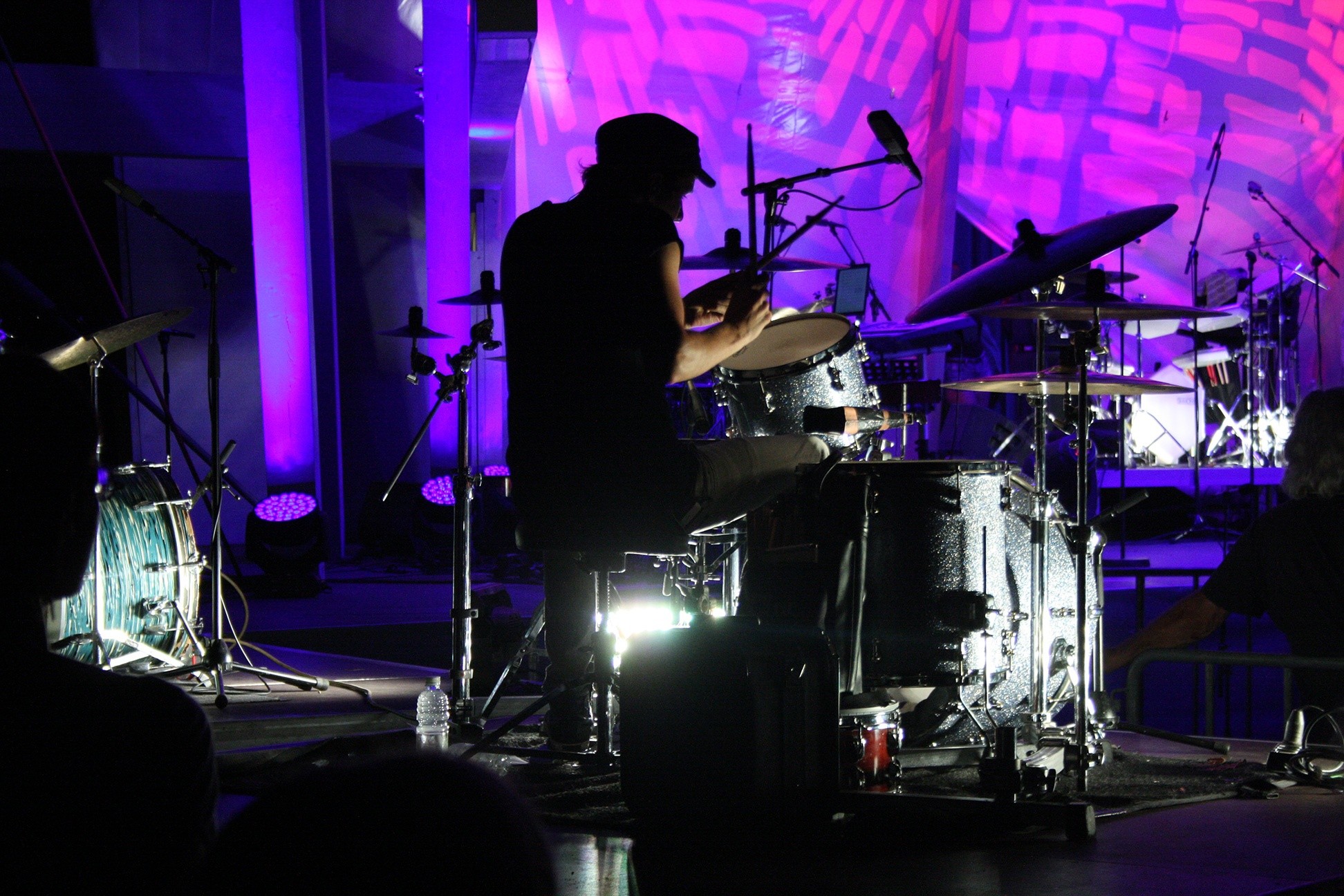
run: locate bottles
[416,676,450,752]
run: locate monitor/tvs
[830,262,870,317]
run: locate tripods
[48,179,329,709]
[1168,123,1244,545]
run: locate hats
[592,112,717,192]
[1285,388,1344,468]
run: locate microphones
[866,109,924,182]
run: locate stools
[535,524,666,767]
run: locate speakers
[620,614,842,846]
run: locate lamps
[416,473,456,566]
[244,481,332,598]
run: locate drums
[830,465,1105,754]
[823,455,1012,690]
[42,458,203,666]
[1121,317,1180,340]
[710,309,885,457]
[1244,263,1301,292]
[1173,346,1251,419]
[1194,269,1246,306]
[1187,306,1250,335]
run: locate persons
[0,345,218,896]
[180,749,559,896]
[500,113,828,534]
[1105,387,1344,752]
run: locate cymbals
[677,249,850,273]
[968,286,1232,322]
[1222,234,1300,258]
[901,200,1181,327]
[435,286,504,305]
[374,324,456,340]
[941,364,1198,396]
[41,304,196,373]
